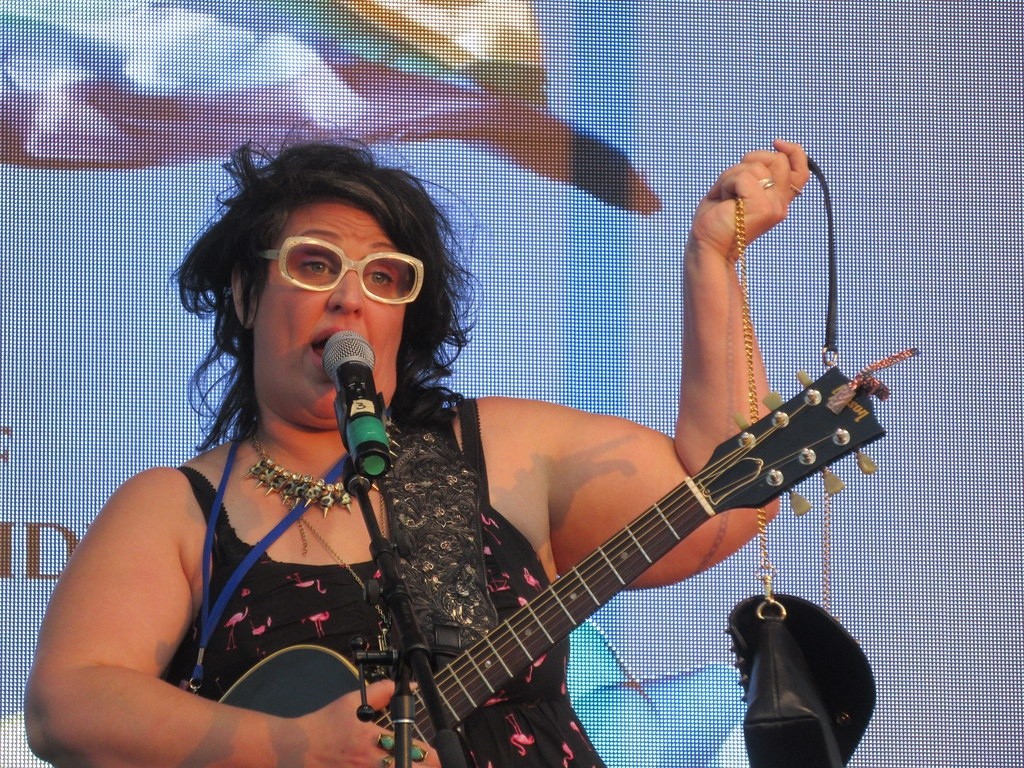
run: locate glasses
[257,236,424,305]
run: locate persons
[24,138,810,768]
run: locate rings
[759,178,775,190]
[790,184,803,196]
[376,733,428,768]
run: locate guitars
[215,363,890,768]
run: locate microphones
[321,330,392,480]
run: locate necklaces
[244,431,379,519]
[277,487,392,651]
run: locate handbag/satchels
[728,593,876,768]
